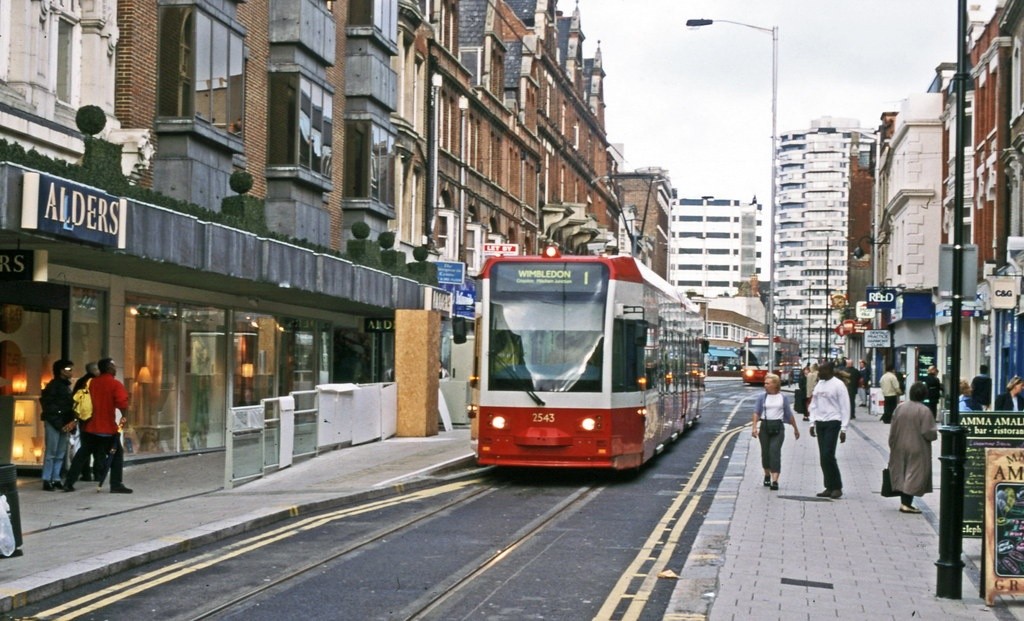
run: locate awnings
[708,349,738,357]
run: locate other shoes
[110,484,133,494]
[898,504,922,513]
[817,487,843,498]
[62,482,75,492]
[803,416,810,422]
[764,475,771,486]
[80,473,91,481]
[770,482,778,490]
[51,481,64,491]
[42,480,55,491]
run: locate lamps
[11,374,27,396]
[137,367,153,425]
[242,360,254,378]
[0,303,25,333]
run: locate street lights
[687,18,776,374]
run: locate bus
[478,245,709,469]
[743,336,800,383]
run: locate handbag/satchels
[73,378,93,421]
[881,463,901,497]
[765,420,780,435]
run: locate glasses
[64,367,72,371]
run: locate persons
[995,376,1024,412]
[879,363,992,424]
[888,382,937,513]
[799,356,870,422]
[38,357,133,493]
[546,332,587,364]
[707,362,739,371]
[809,361,851,498]
[752,374,800,490]
